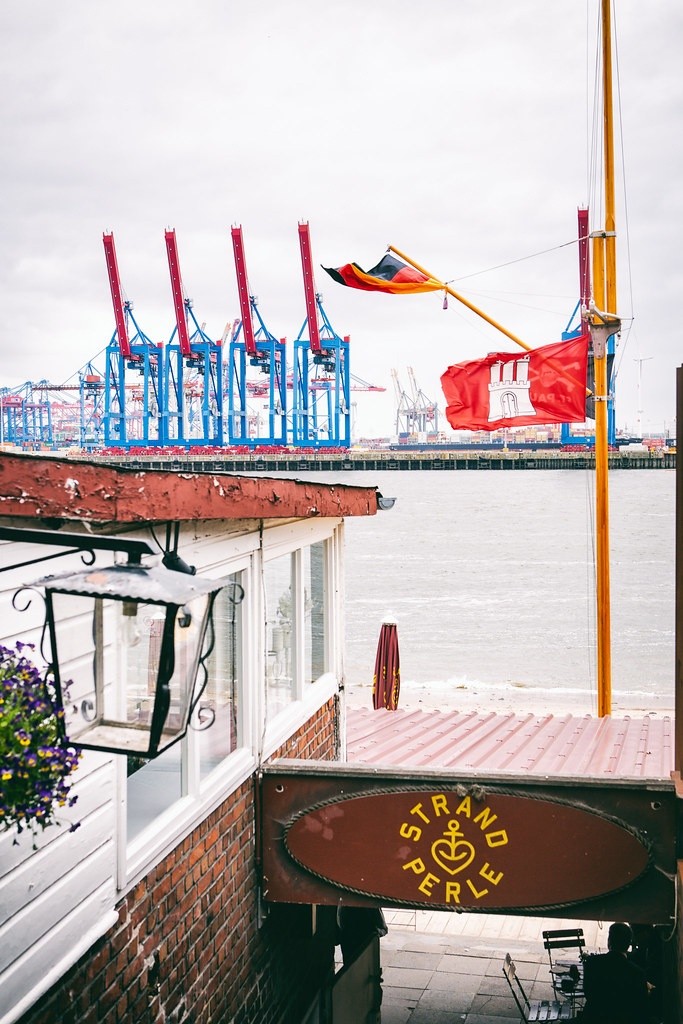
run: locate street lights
[633,357,653,438]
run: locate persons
[582,922,648,1024]
[338,904,388,1024]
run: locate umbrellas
[149,612,166,697]
[372,610,401,711]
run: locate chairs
[502,952,570,1024]
[542,928,587,1018]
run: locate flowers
[0,638,83,851]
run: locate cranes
[390,366,439,435]
[0,220,387,456]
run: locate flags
[320,255,444,294]
[440,334,587,432]
